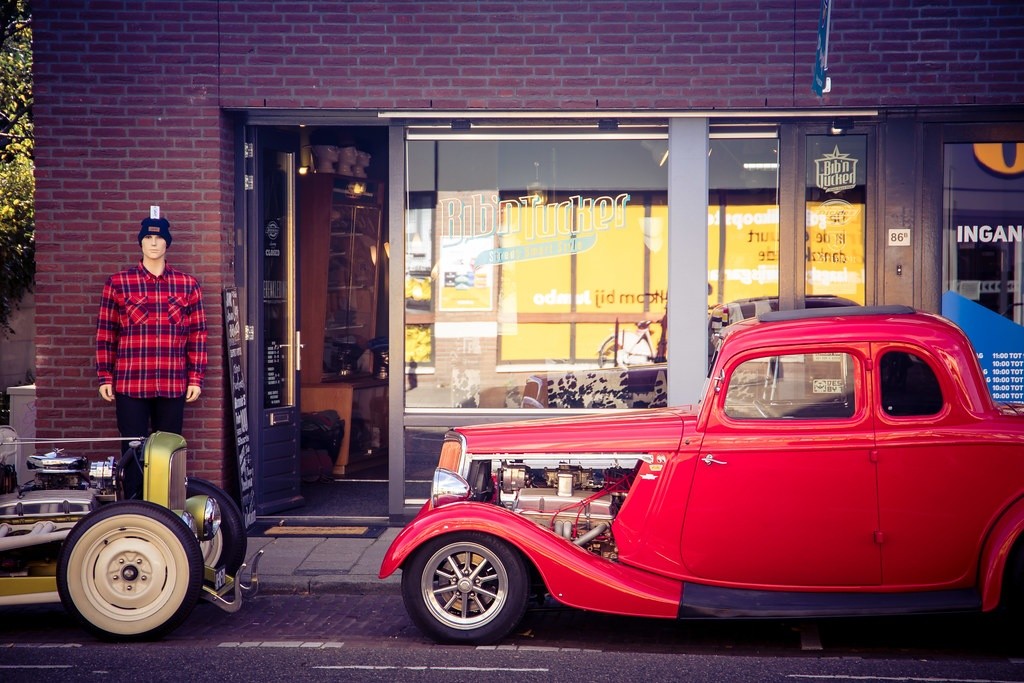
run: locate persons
[94,217,208,501]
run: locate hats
[138,217,172,249]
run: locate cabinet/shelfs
[300,172,384,384]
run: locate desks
[299,379,390,477]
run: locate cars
[707,294,862,369]
[376,302,1024,659]
[0,424,265,646]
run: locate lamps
[597,119,620,130]
[832,117,855,130]
[449,118,471,131]
